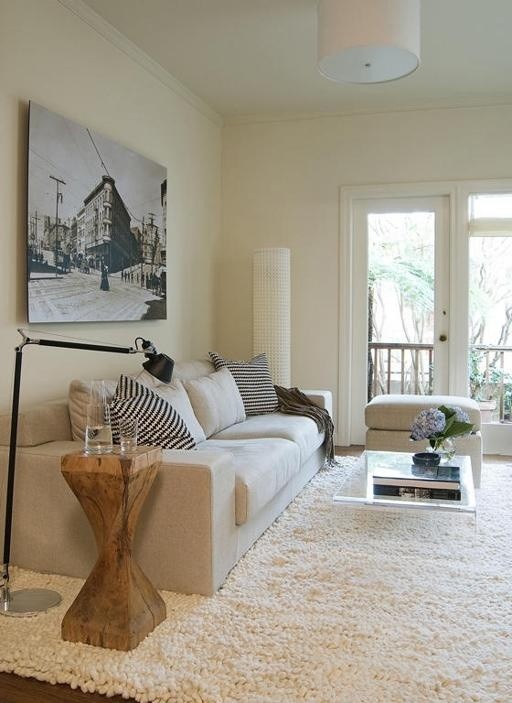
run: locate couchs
[0,356,333,598]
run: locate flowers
[409,405,478,461]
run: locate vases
[424,438,455,464]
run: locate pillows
[105,374,197,452]
[68,379,117,442]
[135,370,168,393]
[152,378,207,444]
[185,367,247,440]
[207,351,278,416]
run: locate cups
[119,416,138,453]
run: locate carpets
[0,458,512,702]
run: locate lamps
[1,329,174,618]
[253,249,292,389]
[315,0,421,85]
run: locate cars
[55,250,76,268]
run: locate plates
[413,452,440,466]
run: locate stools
[364,394,482,488]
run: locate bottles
[86,379,113,453]
